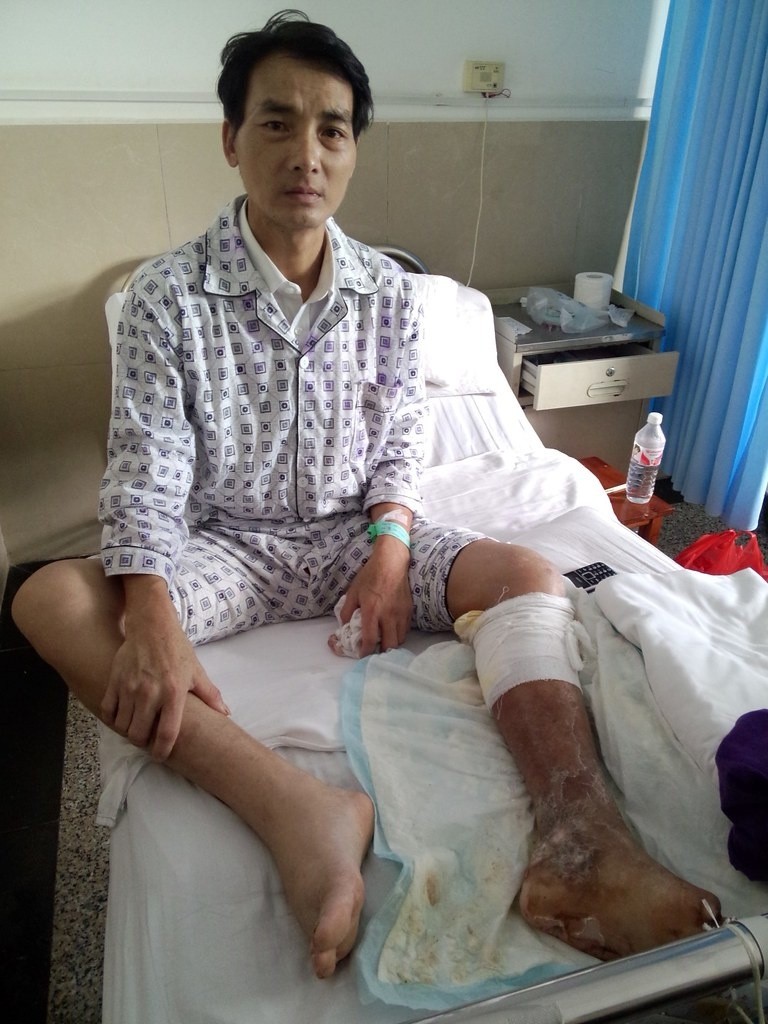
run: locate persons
[11,9,725,979]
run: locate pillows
[103,272,458,388]
[426,286,498,398]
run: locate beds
[94,243,768,1024]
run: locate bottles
[628,411,665,503]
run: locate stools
[577,455,675,547]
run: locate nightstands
[481,284,680,474]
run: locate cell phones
[562,562,618,593]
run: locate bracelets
[367,521,411,549]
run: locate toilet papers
[573,272,614,311]
